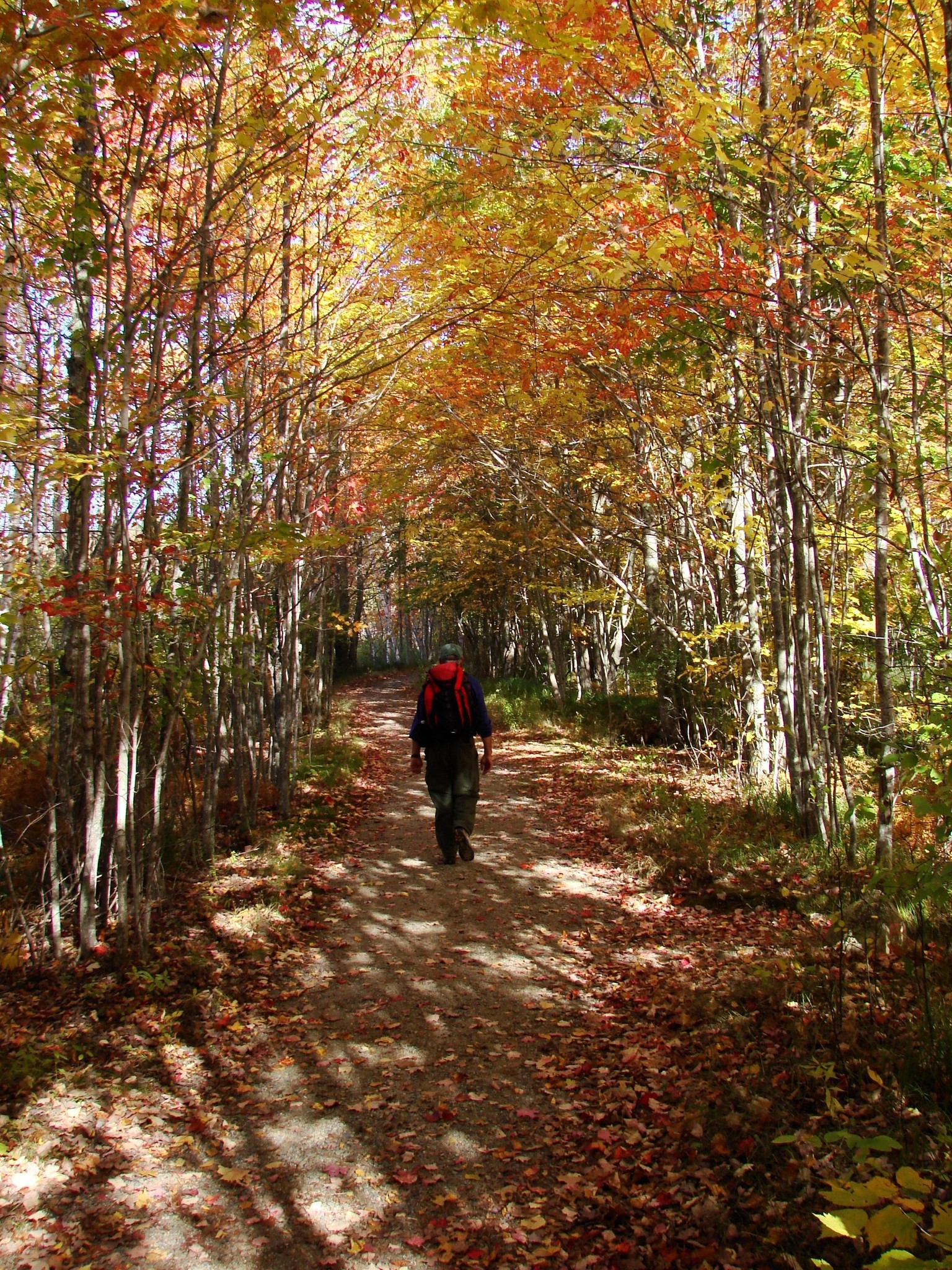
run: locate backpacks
[424,667,472,734]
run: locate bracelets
[411,754,419,758]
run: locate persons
[408,643,493,865]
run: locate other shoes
[453,828,474,861]
[442,856,456,864]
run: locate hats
[440,644,463,660]
